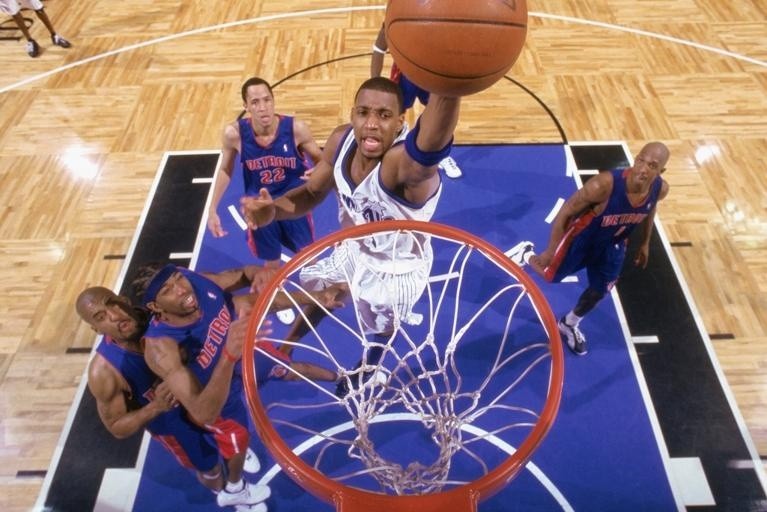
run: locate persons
[236,75,462,395]
[129,260,287,507]
[0,1,70,59]
[501,140,669,358]
[206,74,325,270]
[74,281,346,512]
[368,17,463,180]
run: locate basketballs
[385,0,527,97]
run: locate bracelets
[221,344,236,363]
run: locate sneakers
[557,315,590,357]
[503,240,535,269]
[275,306,296,326]
[27,39,37,57]
[243,446,261,474]
[52,34,71,48]
[334,362,388,399]
[216,481,271,512]
[439,157,464,180]
